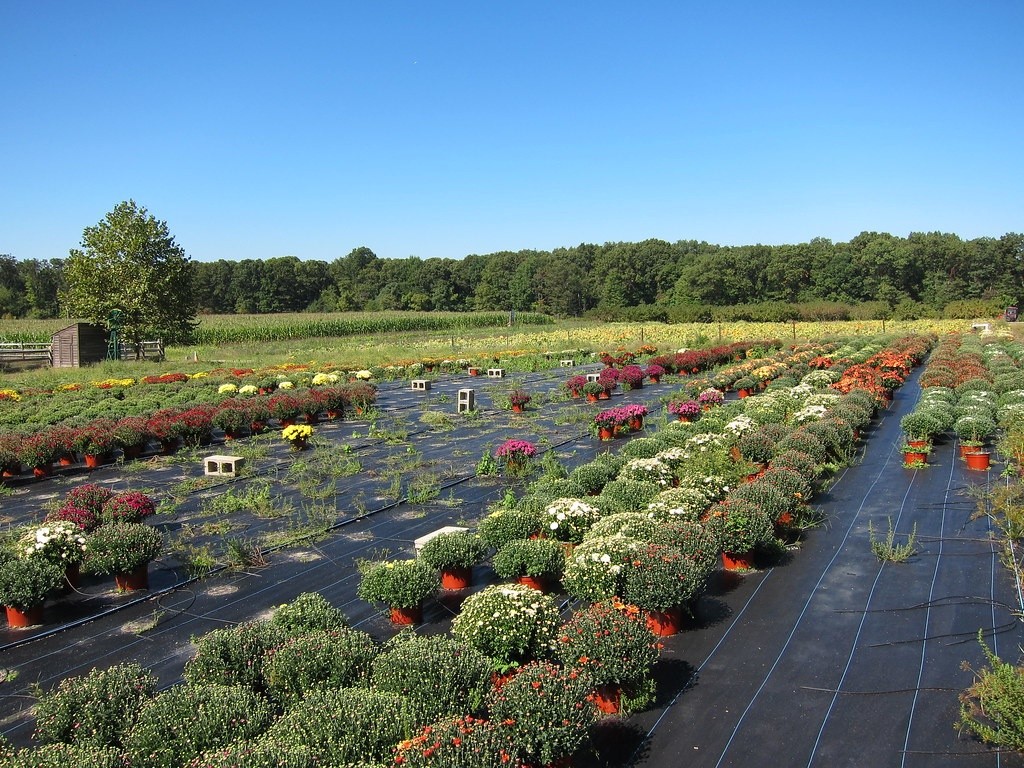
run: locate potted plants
[0,332,1024,768]
